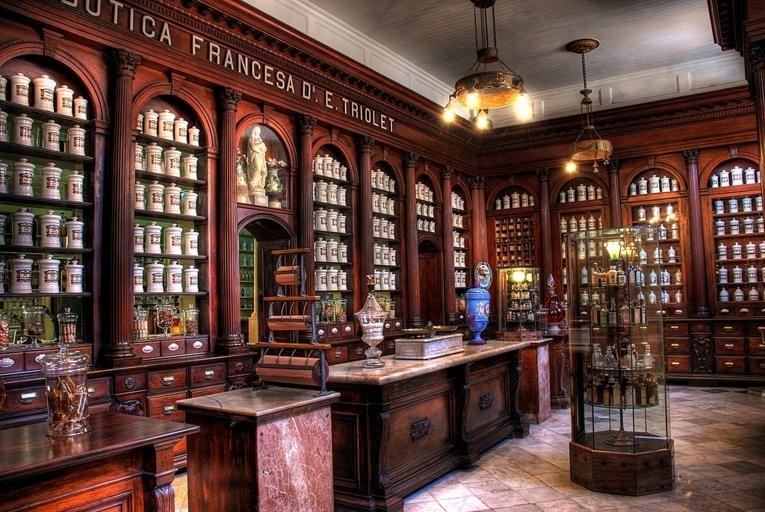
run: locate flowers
[266,155,288,168]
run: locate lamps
[442,1,533,130]
[561,37,615,174]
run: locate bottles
[743,217,753,233]
[581,266,588,287]
[158,110,175,140]
[0,75,7,101]
[591,296,647,327]
[717,243,727,260]
[638,291,645,304]
[147,181,164,212]
[388,198,395,216]
[675,290,682,303]
[66,217,85,248]
[388,221,395,239]
[728,197,738,213]
[316,180,327,203]
[734,286,744,301]
[577,184,587,202]
[511,192,520,208]
[732,242,742,259]
[338,270,347,290]
[373,269,380,290]
[460,270,467,287]
[529,195,535,206]
[371,170,376,188]
[588,214,596,230]
[671,223,679,239]
[454,270,460,287]
[181,189,198,216]
[591,263,645,286]
[668,246,676,263]
[39,210,62,247]
[664,290,670,303]
[380,194,388,214]
[598,216,602,229]
[496,198,501,210]
[718,266,728,283]
[560,191,566,203]
[338,186,347,206]
[41,120,61,151]
[729,218,739,234]
[389,272,396,290]
[381,245,389,266]
[743,196,753,212]
[11,208,35,247]
[66,260,84,294]
[327,209,337,232]
[596,187,602,199]
[40,342,90,438]
[651,205,660,220]
[503,195,510,209]
[761,267,765,281]
[630,183,637,195]
[184,266,199,292]
[134,304,150,340]
[337,213,346,233]
[155,304,174,339]
[638,177,647,194]
[719,287,729,301]
[560,216,567,234]
[649,175,660,194]
[327,239,338,262]
[758,240,765,256]
[428,205,434,217]
[323,154,333,177]
[0,108,9,142]
[459,215,464,228]
[10,72,31,106]
[731,166,743,186]
[745,167,755,184]
[666,203,673,217]
[340,165,348,181]
[461,199,465,210]
[567,187,575,202]
[457,196,461,210]
[376,168,384,190]
[68,125,86,156]
[184,229,199,255]
[315,208,327,231]
[423,219,429,231]
[0,262,6,294]
[587,374,658,405]
[133,263,144,292]
[646,225,654,240]
[416,202,421,215]
[675,269,682,286]
[37,255,60,294]
[578,216,587,231]
[165,182,181,214]
[146,260,164,292]
[134,181,146,210]
[0,160,8,193]
[182,304,200,336]
[74,96,88,120]
[569,216,578,232]
[336,300,347,322]
[428,220,435,232]
[733,265,742,282]
[710,173,719,188]
[164,147,181,177]
[0,215,8,245]
[454,212,459,227]
[327,181,338,205]
[373,244,381,265]
[164,223,182,255]
[417,182,425,200]
[239,236,255,312]
[421,204,428,216]
[314,266,327,291]
[638,206,646,221]
[577,240,586,260]
[13,158,35,197]
[755,216,764,232]
[338,243,348,263]
[12,254,33,293]
[589,240,596,257]
[747,265,757,282]
[663,270,670,285]
[592,343,654,369]
[460,235,465,248]
[183,154,198,180]
[429,190,433,202]
[135,142,143,170]
[326,267,338,289]
[425,185,429,201]
[316,238,327,262]
[634,225,642,241]
[134,223,144,253]
[746,241,756,258]
[755,194,763,211]
[67,171,85,202]
[661,176,670,192]
[13,114,34,147]
[715,219,725,235]
[165,261,183,292]
[756,171,760,183]
[389,178,395,192]
[315,155,323,176]
[671,177,678,191]
[33,75,56,113]
[145,221,161,253]
[136,114,144,133]
[416,218,423,231]
[453,249,459,267]
[451,192,457,209]
[145,142,163,173]
[389,248,396,267]
[188,126,200,147]
[42,163,62,200]
[332,159,340,179]
[372,191,380,213]
[22,298,47,339]
[57,307,78,342]
[639,249,647,264]
[379,218,388,238]
[714,198,724,215]
[415,183,418,199]
[654,247,663,263]
[649,269,657,286]
[174,117,188,145]
[649,291,656,304]
[380,270,389,290]
[748,286,759,301]
[56,84,74,117]
[144,109,158,137]
[373,217,380,237]
[453,231,460,247]
[587,185,595,200]
[459,251,465,267]
[659,224,666,240]
[383,174,389,191]
[719,169,729,186]
[521,192,528,207]
[452,214,456,226]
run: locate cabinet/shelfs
[311,172,352,293]
[560,199,602,320]
[709,182,762,305]
[490,206,539,267]
[0,99,90,299]
[327,340,536,511]
[632,191,682,306]
[630,308,690,373]
[371,187,401,292]
[135,336,260,472]
[174,386,340,512]
[1,413,200,512]
[134,130,207,298]
[0,342,114,414]
[520,338,554,425]
[450,207,470,289]
[537,330,571,410]
[415,198,436,232]
[715,305,765,376]
[314,318,405,364]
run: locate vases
[266,167,283,195]
